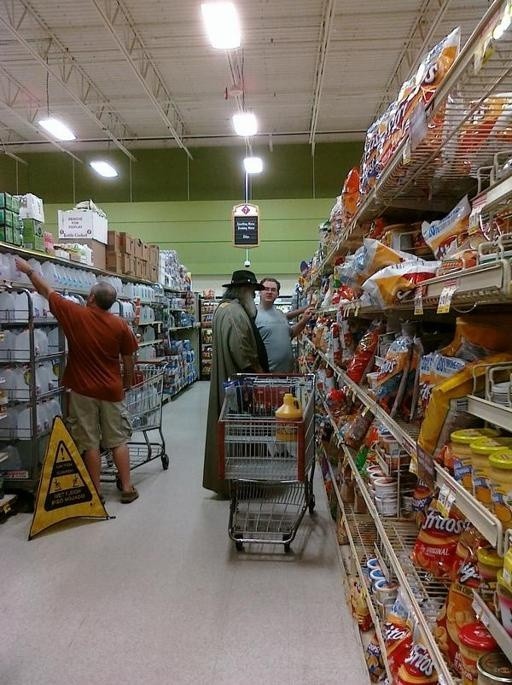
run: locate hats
[221,270,264,291]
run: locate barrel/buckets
[275,393,302,441]
[0,250,156,446]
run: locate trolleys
[216,373,315,553]
[63,360,168,491]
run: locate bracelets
[27,270,34,276]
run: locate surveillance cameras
[244,260,250,267]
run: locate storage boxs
[1,192,22,245]
[107,231,135,255]
[22,219,46,251]
[19,193,46,223]
[57,209,109,245]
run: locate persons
[14,256,140,504]
[254,277,315,375]
[202,271,274,500]
[286,304,314,319]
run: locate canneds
[448,426,512,612]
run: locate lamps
[38,57,78,141]
[197,1,241,49]
[87,136,119,179]
[230,68,256,138]
[243,142,263,175]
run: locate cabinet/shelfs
[293,0,510,685]
[200,296,296,377]
[0,240,200,512]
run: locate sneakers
[120,488,138,504]
